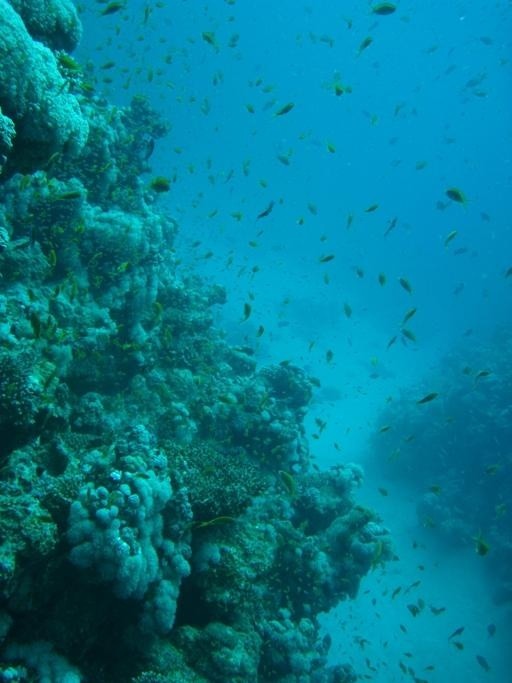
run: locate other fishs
[0,0,512,683]
